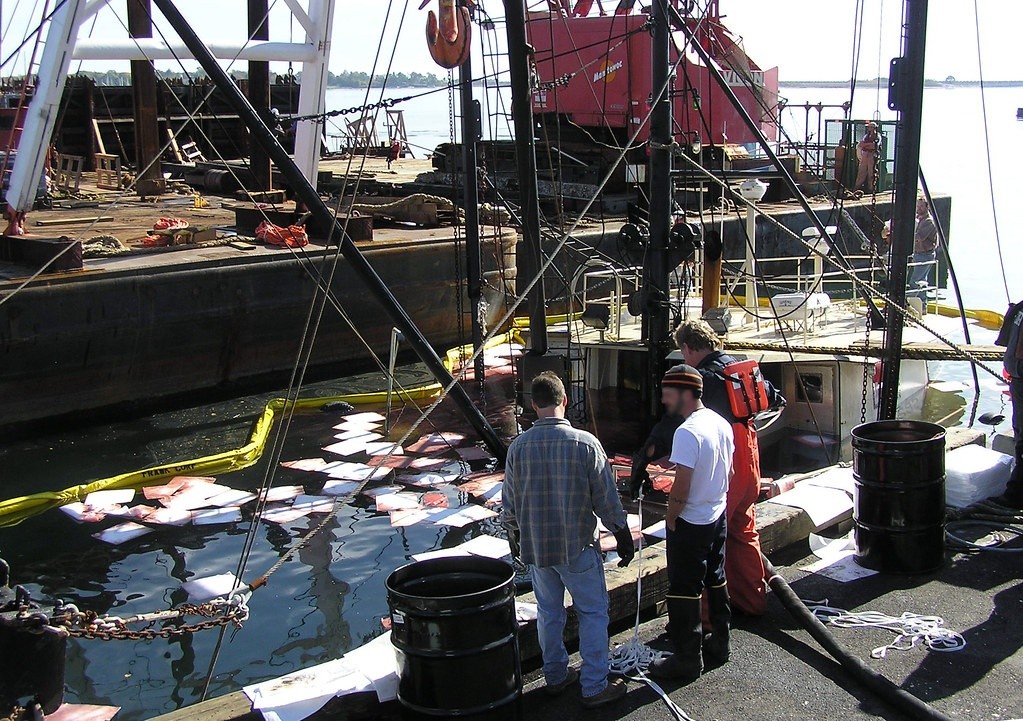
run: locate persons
[907,197,940,316]
[502,371,635,708]
[1002,305,1023,510]
[852,122,882,194]
[835,140,848,185]
[648,364,731,678]
[628,318,787,634]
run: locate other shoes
[705,632,733,660]
[548,668,578,692]
[648,650,702,679]
[581,682,627,706]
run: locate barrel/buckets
[384,555,523,721]
[204,168,237,194]
[850,418,947,572]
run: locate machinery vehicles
[432,0,798,210]
